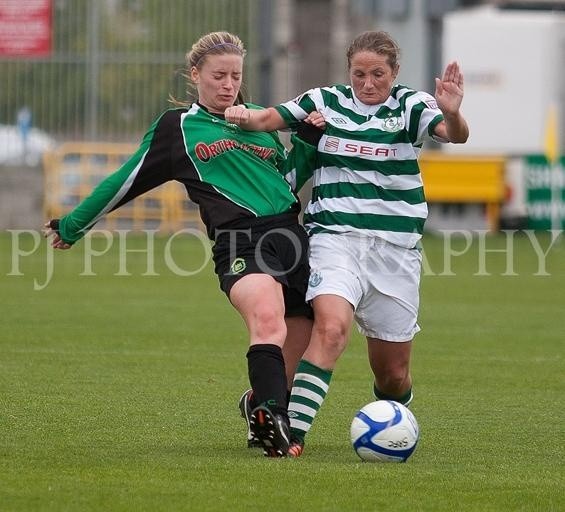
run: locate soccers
[350,399,420,463]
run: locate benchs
[417,153,511,231]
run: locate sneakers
[238,388,304,457]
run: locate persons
[43,32,326,458]
[224,30,469,458]
